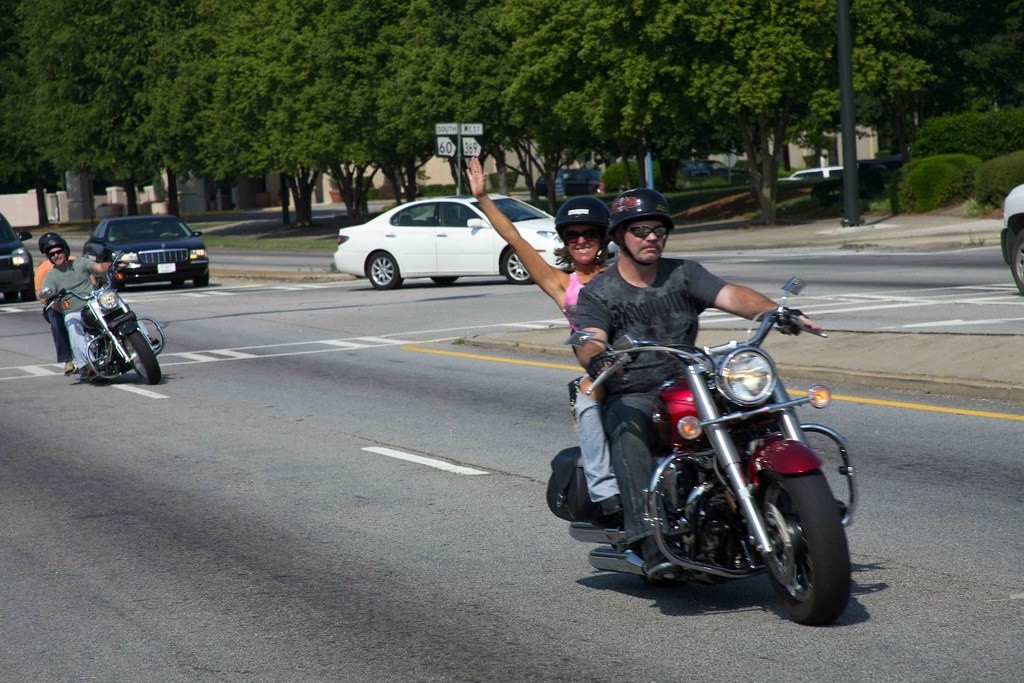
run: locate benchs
[399,215,438,226]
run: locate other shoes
[65,361,75,372]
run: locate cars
[1,215,36,301]
[334,192,620,290]
[534,169,604,196]
[856,151,903,172]
[79,213,209,287]
[777,165,843,180]
[1000,182,1024,294]
[680,157,746,179]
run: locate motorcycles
[568,277,858,626]
[39,250,170,386]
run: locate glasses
[561,228,605,243]
[623,226,668,238]
[49,249,64,256]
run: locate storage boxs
[546,445,602,522]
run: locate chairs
[447,209,465,225]
[110,225,131,241]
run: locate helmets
[555,196,612,236]
[39,233,60,253]
[45,237,68,254]
[606,188,674,239]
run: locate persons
[574,190,822,581]
[34,231,101,367]
[459,157,621,518]
[42,241,116,374]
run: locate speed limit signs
[437,136,460,158]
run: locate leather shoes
[601,495,622,515]
[641,539,671,572]
